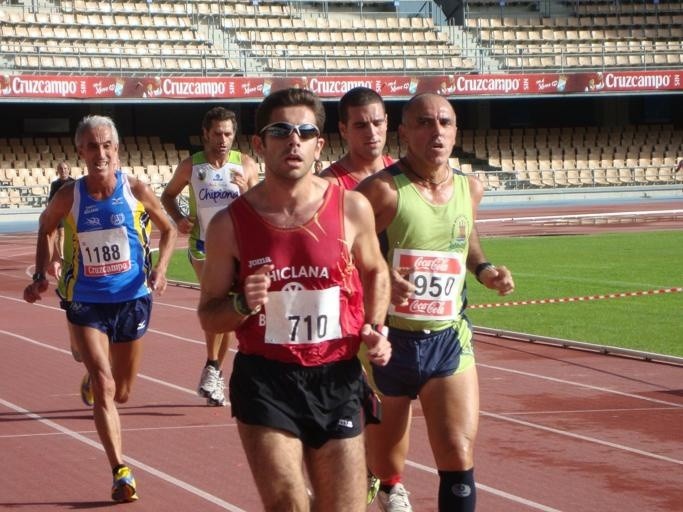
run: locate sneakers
[197,364,227,408]
[111,466,139,503]
[366,470,412,512]
[81,371,96,407]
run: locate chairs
[0,125,683,208]
[0,1,683,74]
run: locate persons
[160,107,260,407]
[47,155,120,406]
[198,88,393,512]
[24,115,178,502]
[49,161,77,258]
[318,87,393,504]
[350,91,514,512]
[313,161,323,176]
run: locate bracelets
[226,292,251,317]
[369,320,389,337]
[476,262,495,284]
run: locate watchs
[32,273,46,282]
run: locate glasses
[260,119,321,140]
